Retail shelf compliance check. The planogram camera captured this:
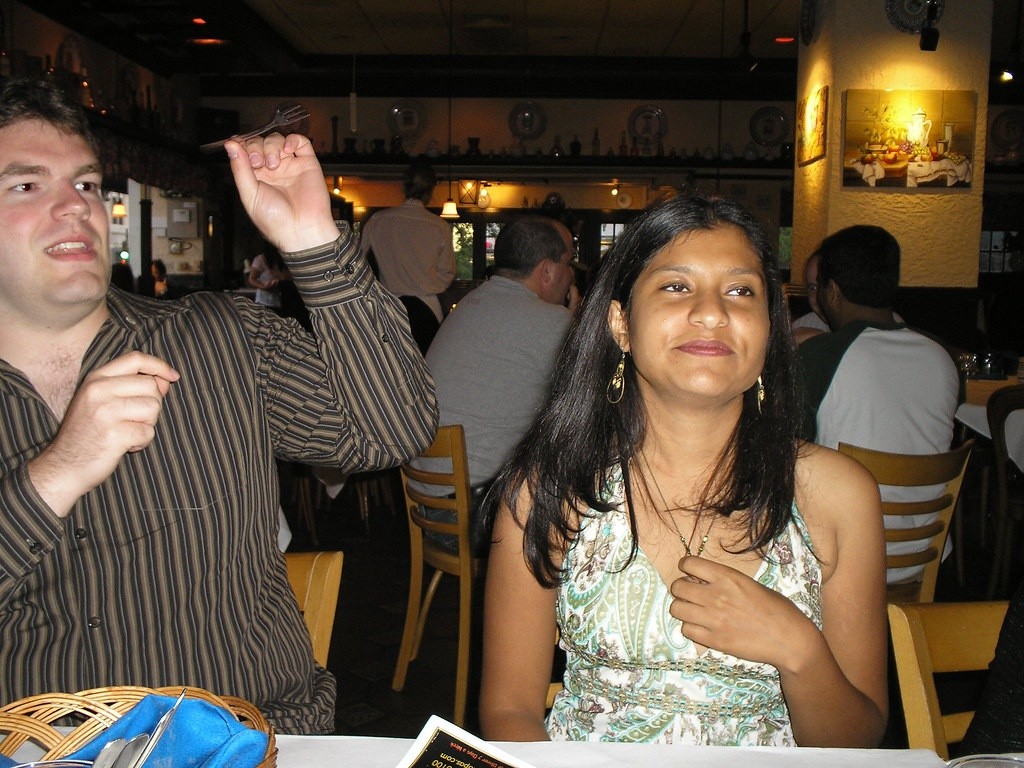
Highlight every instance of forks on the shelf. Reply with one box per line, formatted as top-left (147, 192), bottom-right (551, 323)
top-left (199, 104), bottom-right (311, 152)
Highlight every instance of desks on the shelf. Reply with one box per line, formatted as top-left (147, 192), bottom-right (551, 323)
top-left (0, 734), bottom-right (951, 768)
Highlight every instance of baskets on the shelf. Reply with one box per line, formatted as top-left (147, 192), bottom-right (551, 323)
top-left (0, 687), bottom-right (279, 768)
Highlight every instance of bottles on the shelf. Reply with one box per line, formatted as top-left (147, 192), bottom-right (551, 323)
top-left (670, 147), bottom-right (676, 160)
top-left (571, 134), bottom-right (581, 158)
top-left (552, 135), bottom-right (561, 162)
top-left (619, 130), bottom-right (628, 159)
top-left (959, 351), bottom-right (980, 397)
top-left (655, 134), bottom-right (663, 161)
top-left (591, 128), bottom-right (600, 158)
top-left (426, 140), bottom-right (440, 158)
top-left (694, 147), bottom-right (700, 160)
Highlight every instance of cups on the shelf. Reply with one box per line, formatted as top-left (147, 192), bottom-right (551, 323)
top-left (944, 754), bottom-right (1024, 768)
top-left (9, 760), bottom-right (95, 768)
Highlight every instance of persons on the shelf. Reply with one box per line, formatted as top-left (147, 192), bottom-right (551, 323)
top-left (150, 260), bottom-right (178, 299)
top-left (0, 67), bottom-right (443, 737)
top-left (955, 583), bottom-right (1024, 756)
top-left (482, 193), bottom-right (890, 749)
top-left (789, 224), bottom-right (961, 597)
top-left (247, 158), bottom-right (584, 555)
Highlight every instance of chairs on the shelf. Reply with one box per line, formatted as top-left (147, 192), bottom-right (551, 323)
top-left (280, 550), bottom-right (346, 667)
top-left (390, 422), bottom-right (493, 730)
top-left (883, 594), bottom-right (1011, 755)
top-left (835, 438), bottom-right (982, 604)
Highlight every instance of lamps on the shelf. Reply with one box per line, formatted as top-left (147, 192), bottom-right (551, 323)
top-left (110, 192), bottom-right (128, 219)
top-left (442, 0), bottom-right (460, 220)
top-left (735, 0), bottom-right (760, 72)
top-left (331, 176), bottom-right (344, 195)
top-left (610, 182), bottom-right (622, 196)
top-left (918, 0), bottom-right (941, 52)
top-left (346, 50), bottom-right (360, 133)
top-left (478, 182), bottom-right (495, 208)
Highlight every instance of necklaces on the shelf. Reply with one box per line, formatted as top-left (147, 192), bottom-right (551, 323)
top-left (640, 444), bottom-right (725, 583)
